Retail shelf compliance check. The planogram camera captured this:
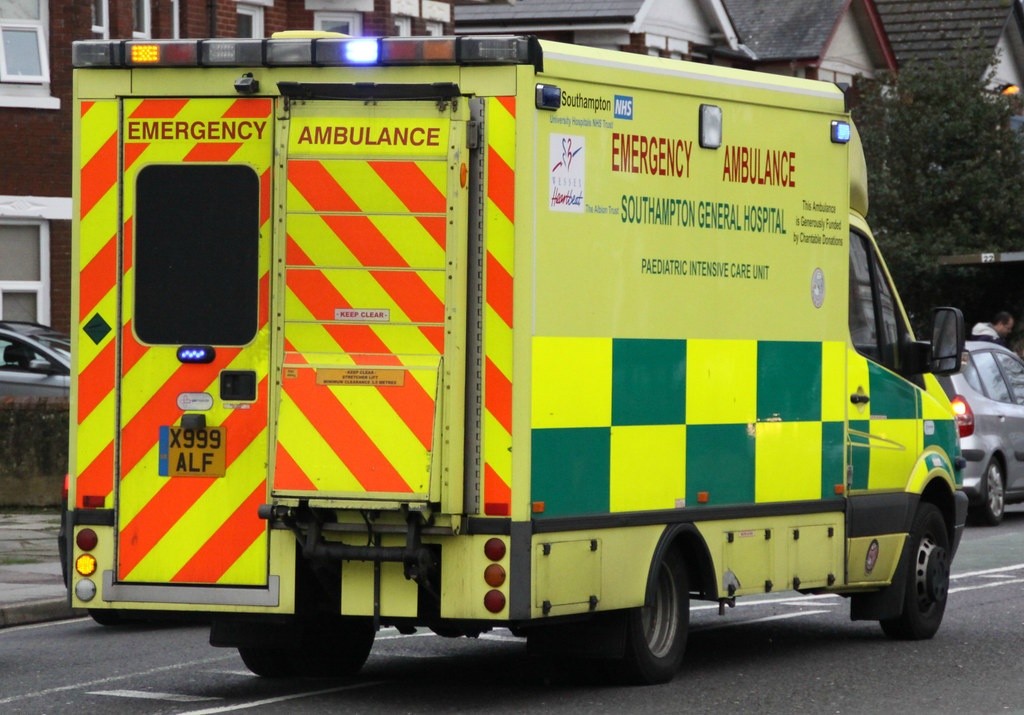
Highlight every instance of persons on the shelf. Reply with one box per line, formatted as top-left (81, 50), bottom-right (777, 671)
top-left (970, 310), bottom-right (1014, 345)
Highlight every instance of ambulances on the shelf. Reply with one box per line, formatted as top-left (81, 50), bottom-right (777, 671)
top-left (58, 21), bottom-right (971, 687)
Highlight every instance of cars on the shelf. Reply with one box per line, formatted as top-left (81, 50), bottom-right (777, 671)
top-left (912, 340), bottom-right (1023, 528)
top-left (1, 318), bottom-right (71, 401)
